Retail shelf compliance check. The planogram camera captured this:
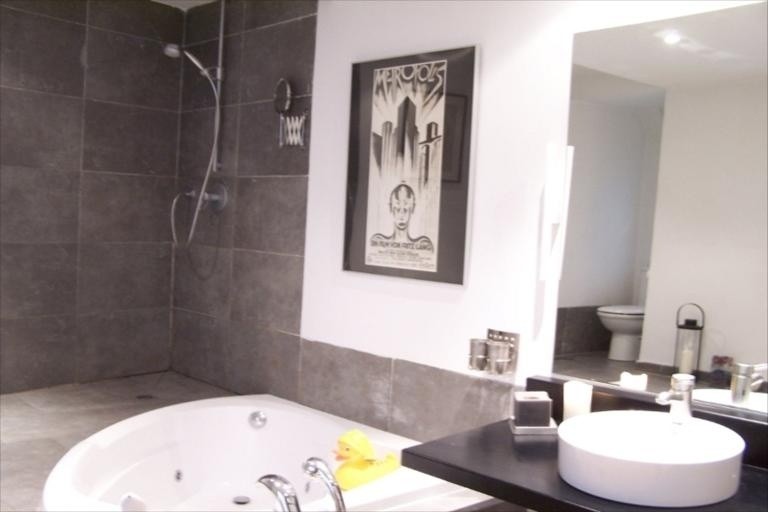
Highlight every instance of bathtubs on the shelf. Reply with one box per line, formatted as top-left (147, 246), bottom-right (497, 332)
top-left (42, 393), bottom-right (495, 512)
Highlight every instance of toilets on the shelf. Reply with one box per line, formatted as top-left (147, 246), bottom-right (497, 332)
top-left (597, 268), bottom-right (650, 362)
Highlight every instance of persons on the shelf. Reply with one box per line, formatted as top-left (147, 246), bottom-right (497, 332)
top-left (369, 185), bottom-right (434, 253)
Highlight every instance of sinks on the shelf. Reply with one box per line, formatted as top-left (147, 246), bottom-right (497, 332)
top-left (558, 411), bottom-right (747, 508)
top-left (692, 388), bottom-right (768, 426)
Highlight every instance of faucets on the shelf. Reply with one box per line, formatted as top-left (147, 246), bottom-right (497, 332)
top-left (730, 364), bottom-right (759, 402)
top-left (653, 373), bottom-right (695, 418)
top-left (301, 456), bottom-right (346, 512)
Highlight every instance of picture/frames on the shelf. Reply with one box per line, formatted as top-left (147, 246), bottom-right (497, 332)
top-left (343, 44), bottom-right (479, 284)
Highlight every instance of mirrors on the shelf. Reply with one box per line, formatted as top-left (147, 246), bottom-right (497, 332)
top-left (550, 1), bottom-right (768, 413)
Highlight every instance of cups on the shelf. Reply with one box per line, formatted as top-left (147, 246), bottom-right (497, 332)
top-left (486, 341), bottom-right (510, 375)
top-left (470, 339), bottom-right (488, 370)
top-left (562, 380), bottom-right (593, 421)
